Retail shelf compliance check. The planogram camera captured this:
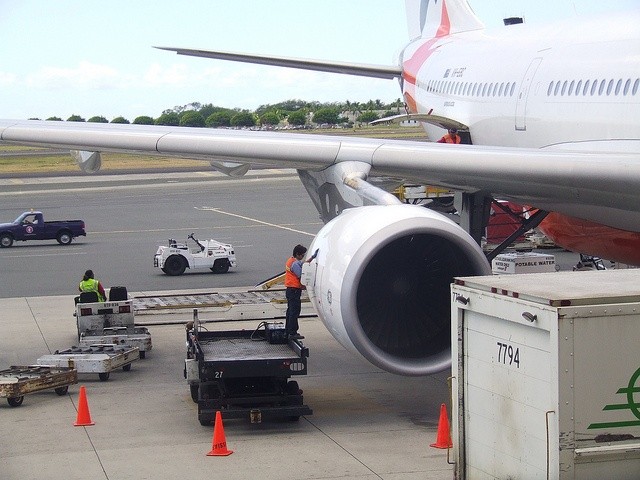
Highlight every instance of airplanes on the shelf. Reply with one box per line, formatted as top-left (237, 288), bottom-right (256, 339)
top-left (1, 1), bottom-right (639, 377)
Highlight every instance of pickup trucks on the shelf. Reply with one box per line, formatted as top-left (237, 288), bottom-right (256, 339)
top-left (0, 209), bottom-right (86, 248)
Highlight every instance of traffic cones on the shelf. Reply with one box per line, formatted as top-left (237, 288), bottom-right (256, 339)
top-left (429, 403), bottom-right (453, 449)
top-left (206, 411), bottom-right (233, 456)
top-left (72, 385), bottom-right (95, 426)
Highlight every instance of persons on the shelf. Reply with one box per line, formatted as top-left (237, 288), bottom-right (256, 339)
top-left (437, 128), bottom-right (461, 144)
top-left (78, 269), bottom-right (106, 302)
top-left (283, 244), bottom-right (319, 341)
top-left (25, 216), bottom-right (39, 225)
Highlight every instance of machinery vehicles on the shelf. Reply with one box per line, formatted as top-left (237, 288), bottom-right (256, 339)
top-left (183, 310), bottom-right (312, 425)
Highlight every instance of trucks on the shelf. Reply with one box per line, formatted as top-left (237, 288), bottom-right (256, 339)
top-left (446, 267), bottom-right (640, 480)
